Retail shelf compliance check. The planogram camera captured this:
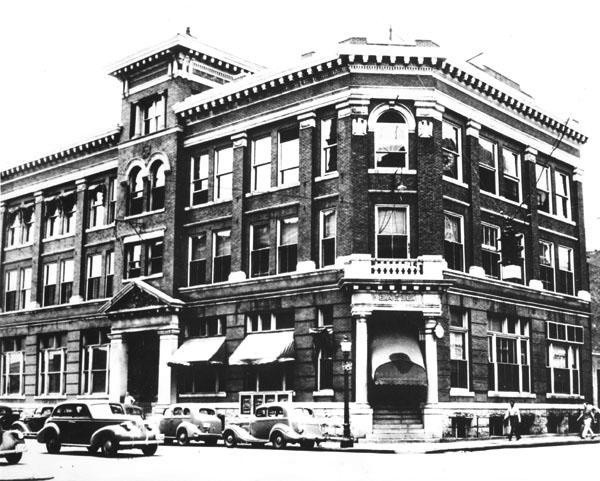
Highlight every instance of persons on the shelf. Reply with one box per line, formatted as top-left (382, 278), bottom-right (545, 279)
top-left (504, 400), bottom-right (522, 441)
top-left (576, 401), bottom-right (597, 439)
top-left (124, 392), bottom-right (136, 405)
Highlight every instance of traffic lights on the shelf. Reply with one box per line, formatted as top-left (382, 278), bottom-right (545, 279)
top-left (496, 228), bottom-right (507, 266)
top-left (508, 228), bottom-right (525, 266)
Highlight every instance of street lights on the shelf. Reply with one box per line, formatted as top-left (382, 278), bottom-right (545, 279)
top-left (339, 335), bottom-right (354, 448)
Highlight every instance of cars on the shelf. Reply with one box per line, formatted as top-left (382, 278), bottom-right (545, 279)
top-left (0, 399), bottom-right (56, 465)
top-left (222, 401), bottom-right (328, 450)
top-left (158, 403), bottom-right (223, 446)
top-left (35, 399), bottom-right (165, 458)
